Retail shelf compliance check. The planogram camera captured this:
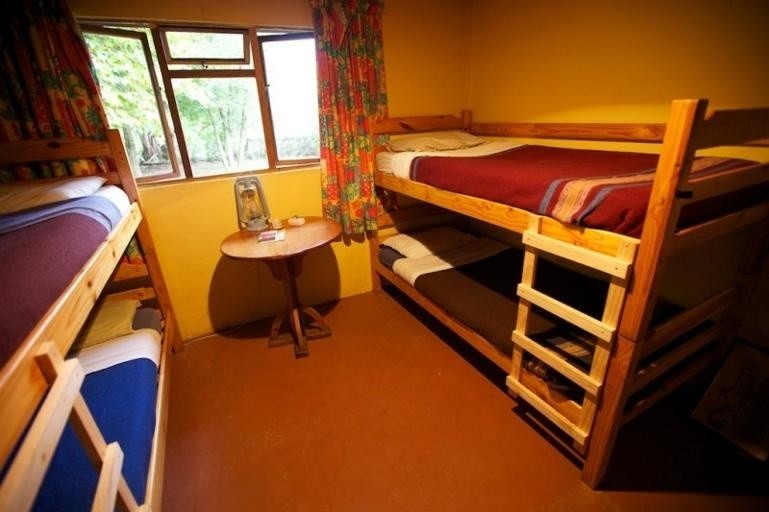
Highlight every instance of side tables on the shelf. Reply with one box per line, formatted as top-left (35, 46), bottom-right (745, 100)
top-left (220, 215), bottom-right (345, 359)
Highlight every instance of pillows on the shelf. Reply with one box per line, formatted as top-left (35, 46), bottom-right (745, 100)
top-left (383, 129), bottom-right (488, 153)
top-left (67, 297), bottom-right (142, 345)
top-left (1, 174), bottom-right (108, 215)
top-left (380, 225), bottom-right (478, 260)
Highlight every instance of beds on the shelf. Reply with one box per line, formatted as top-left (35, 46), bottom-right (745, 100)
top-left (0, 123), bottom-right (187, 512)
top-left (367, 93), bottom-right (760, 490)
